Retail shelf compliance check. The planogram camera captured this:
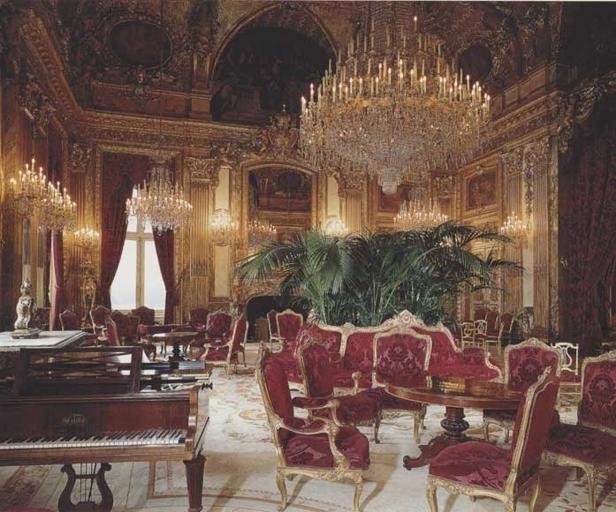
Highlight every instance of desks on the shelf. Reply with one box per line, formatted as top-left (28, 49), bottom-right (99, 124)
top-left (384, 372), bottom-right (527, 472)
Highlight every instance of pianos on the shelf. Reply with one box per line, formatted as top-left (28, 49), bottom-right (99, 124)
top-left (0, 345), bottom-right (213, 512)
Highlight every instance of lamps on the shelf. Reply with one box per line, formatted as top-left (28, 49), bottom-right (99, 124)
top-left (0, 102), bottom-right (278, 242)
top-left (294, 0), bottom-right (524, 240)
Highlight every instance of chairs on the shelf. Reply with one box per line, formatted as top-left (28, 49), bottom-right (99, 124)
top-left (260, 307), bottom-right (585, 405)
top-left (424, 359), bottom-right (562, 512)
top-left (0, 299), bottom-right (247, 385)
top-left (536, 349), bottom-right (616, 512)
top-left (254, 338), bottom-right (372, 511)
top-left (482, 336), bottom-right (560, 445)
top-left (296, 338), bottom-right (384, 446)
top-left (350, 324), bottom-right (435, 442)
